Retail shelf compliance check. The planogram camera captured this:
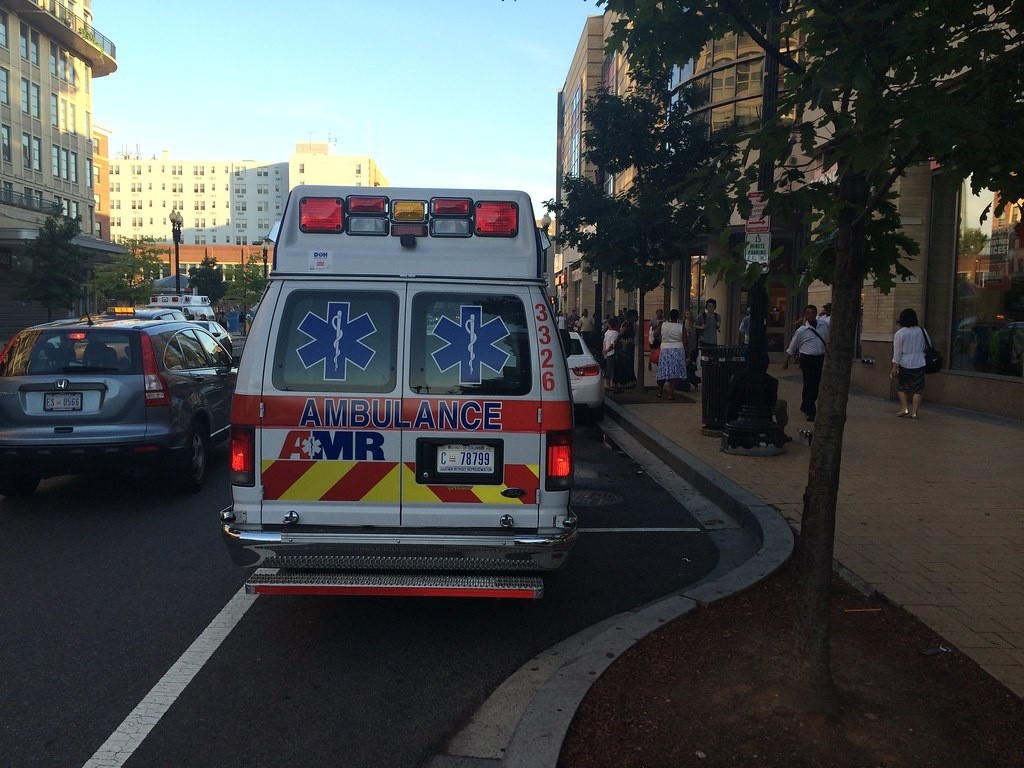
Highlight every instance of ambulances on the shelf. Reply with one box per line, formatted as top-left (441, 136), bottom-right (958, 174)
top-left (219, 186), bottom-right (575, 600)
top-left (150, 293), bottom-right (216, 322)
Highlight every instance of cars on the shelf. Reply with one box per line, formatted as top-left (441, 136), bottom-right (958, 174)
top-left (189, 320), bottom-right (233, 357)
top-left (568, 331), bottom-right (605, 424)
top-left (99, 306), bottom-right (187, 321)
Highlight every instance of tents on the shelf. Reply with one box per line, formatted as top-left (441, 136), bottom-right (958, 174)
top-left (154, 274), bottom-right (190, 288)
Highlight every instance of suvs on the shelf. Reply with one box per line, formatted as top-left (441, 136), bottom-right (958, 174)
top-left (0, 317), bottom-right (242, 501)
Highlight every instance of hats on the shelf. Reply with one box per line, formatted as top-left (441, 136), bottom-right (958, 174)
top-left (823, 303), bottom-right (831, 309)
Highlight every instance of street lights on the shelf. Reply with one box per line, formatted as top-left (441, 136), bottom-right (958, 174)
top-left (541, 213), bottom-right (551, 276)
top-left (261, 239), bottom-right (268, 279)
top-left (168, 210), bottom-right (184, 296)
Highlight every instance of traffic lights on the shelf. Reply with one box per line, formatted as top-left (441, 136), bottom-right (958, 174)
top-left (550, 296), bottom-right (555, 305)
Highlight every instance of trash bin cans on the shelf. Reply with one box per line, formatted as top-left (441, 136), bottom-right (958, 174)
top-left (696, 344), bottom-right (755, 437)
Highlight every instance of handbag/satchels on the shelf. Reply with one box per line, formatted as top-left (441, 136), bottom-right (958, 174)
top-left (924, 348), bottom-right (944, 374)
top-left (649, 327), bottom-right (655, 345)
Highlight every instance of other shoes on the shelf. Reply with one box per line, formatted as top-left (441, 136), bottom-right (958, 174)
top-left (656, 390), bottom-right (662, 397)
top-left (647, 359), bottom-right (652, 371)
top-left (897, 408), bottom-right (909, 417)
top-left (911, 414), bottom-right (919, 419)
top-left (605, 383), bottom-right (618, 390)
top-left (800, 407), bottom-right (815, 421)
top-left (668, 394), bottom-right (674, 399)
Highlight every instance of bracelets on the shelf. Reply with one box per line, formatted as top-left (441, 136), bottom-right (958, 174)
top-left (893, 367), bottom-right (897, 368)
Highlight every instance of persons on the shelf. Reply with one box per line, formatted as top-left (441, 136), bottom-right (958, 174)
top-left (683, 309), bottom-right (699, 366)
top-left (694, 299), bottom-right (721, 347)
top-left (215, 312), bottom-right (227, 331)
top-left (199, 313), bottom-right (214, 321)
top-left (889, 308), bottom-right (933, 419)
top-left (648, 308), bottom-right (668, 371)
top-left (603, 309), bottom-right (638, 386)
top-left (239, 311), bottom-right (245, 336)
top-left (555, 307), bottom-right (638, 391)
top-left (577, 309), bottom-right (596, 352)
top-left (783, 303), bottom-right (833, 422)
top-left (656, 309), bottom-right (688, 400)
top-left (603, 315), bottom-right (620, 391)
top-left (739, 304), bottom-right (752, 345)
